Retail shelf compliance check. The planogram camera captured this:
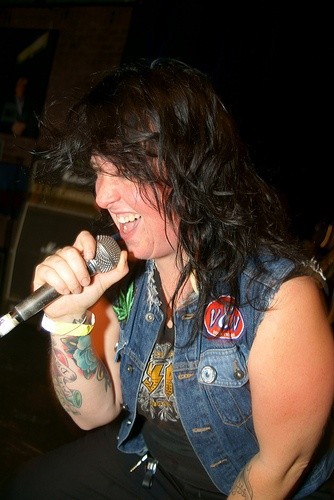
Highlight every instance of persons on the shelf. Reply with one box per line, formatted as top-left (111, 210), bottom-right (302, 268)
top-left (0, 56), bottom-right (334, 500)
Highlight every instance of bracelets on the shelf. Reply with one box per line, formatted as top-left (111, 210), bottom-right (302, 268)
top-left (41, 312), bottom-right (95, 336)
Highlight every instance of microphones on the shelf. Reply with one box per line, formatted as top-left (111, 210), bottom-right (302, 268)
top-left (0, 234), bottom-right (121, 339)
